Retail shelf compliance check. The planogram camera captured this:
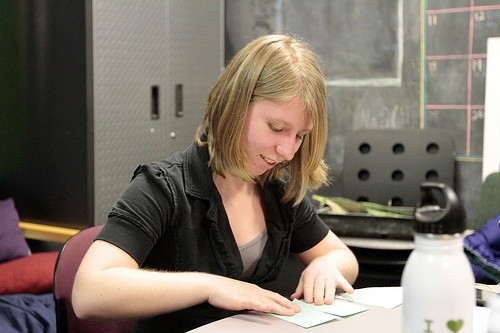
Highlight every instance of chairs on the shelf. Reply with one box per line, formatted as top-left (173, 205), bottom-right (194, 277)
top-left (53, 225), bottom-right (140, 333)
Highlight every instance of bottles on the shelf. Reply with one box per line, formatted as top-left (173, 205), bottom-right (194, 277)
top-left (400, 182), bottom-right (477, 333)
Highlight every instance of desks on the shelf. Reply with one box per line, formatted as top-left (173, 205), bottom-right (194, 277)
top-left (184, 283), bottom-right (499, 333)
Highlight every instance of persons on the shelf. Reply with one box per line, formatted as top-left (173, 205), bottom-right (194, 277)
top-left (70, 34), bottom-right (360, 333)
top-left (464, 213), bottom-right (500, 285)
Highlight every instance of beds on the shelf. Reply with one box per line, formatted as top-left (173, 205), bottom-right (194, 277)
top-left (0, 197), bottom-right (62, 333)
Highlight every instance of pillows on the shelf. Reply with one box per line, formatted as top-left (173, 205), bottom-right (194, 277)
top-left (0, 249), bottom-right (62, 295)
top-left (0, 198), bottom-right (32, 261)
